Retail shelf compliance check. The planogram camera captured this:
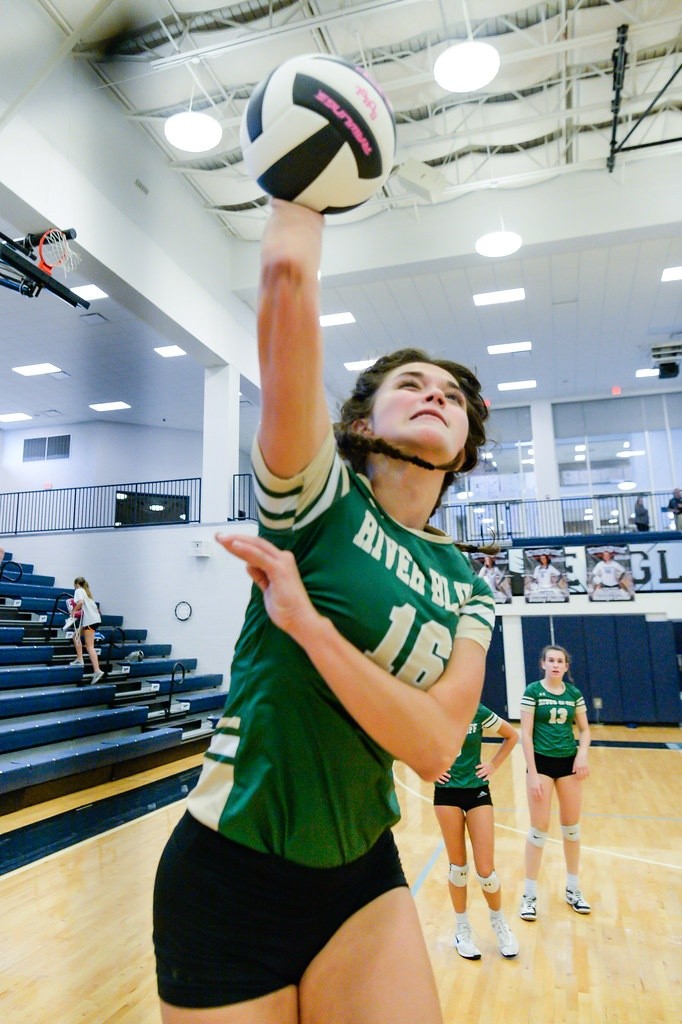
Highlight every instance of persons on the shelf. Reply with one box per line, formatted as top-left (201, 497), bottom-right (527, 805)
top-left (68, 577), bottom-right (105, 686)
top-left (589, 551), bottom-right (634, 602)
top-left (431, 701), bottom-right (521, 959)
top-left (150, 195), bottom-right (496, 1024)
top-left (524, 554), bottom-right (569, 602)
top-left (633, 496), bottom-right (650, 533)
top-left (666, 489), bottom-right (682, 533)
top-left (521, 646), bottom-right (591, 923)
top-left (478, 555), bottom-right (512, 604)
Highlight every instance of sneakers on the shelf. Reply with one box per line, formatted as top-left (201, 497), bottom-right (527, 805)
top-left (492, 914), bottom-right (519, 957)
top-left (565, 886), bottom-right (590, 914)
top-left (519, 894), bottom-right (537, 921)
top-left (452, 922), bottom-right (482, 960)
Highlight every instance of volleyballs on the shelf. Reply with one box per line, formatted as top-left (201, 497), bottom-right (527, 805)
top-left (241, 51), bottom-right (398, 217)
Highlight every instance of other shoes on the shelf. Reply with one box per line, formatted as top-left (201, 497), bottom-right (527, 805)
top-left (63, 618), bottom-right (78, 630)
top-left (91, 670), bottom-right (104, 685)
top-left (69, 658), bottom-right (84, 665)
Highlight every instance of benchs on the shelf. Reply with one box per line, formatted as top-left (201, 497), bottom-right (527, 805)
top-left (0, 628), bottom-right (183, 796)
top-left (0, 553), bottom-right (230, 729)
